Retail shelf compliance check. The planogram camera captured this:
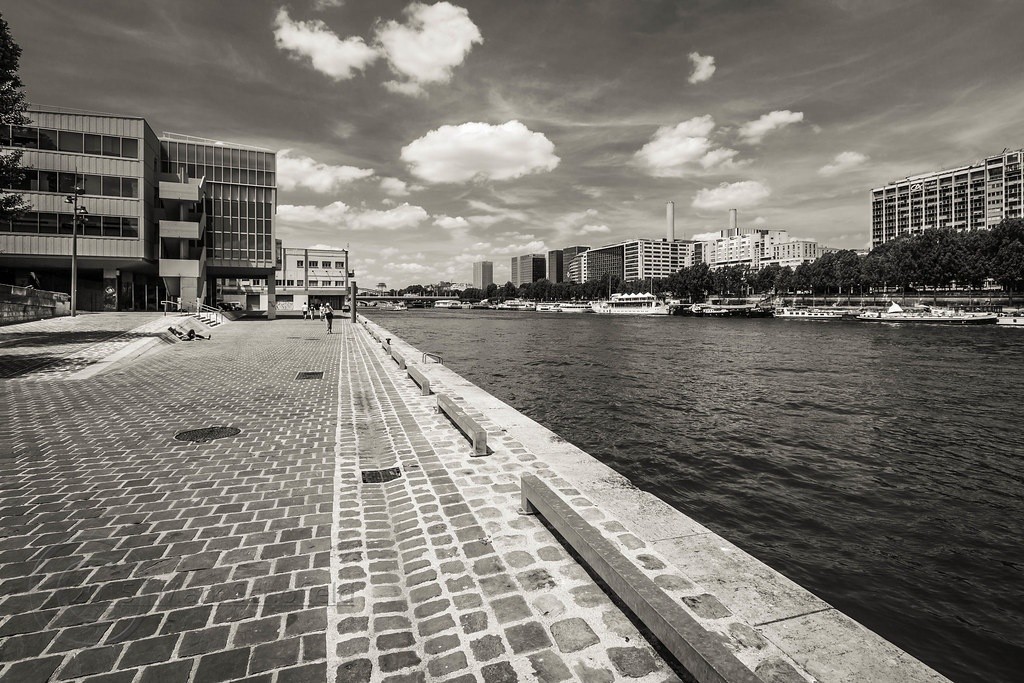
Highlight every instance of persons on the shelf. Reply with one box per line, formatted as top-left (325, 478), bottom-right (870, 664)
top-left (309, 303), bottom-right (316, 320)
top-left (319, 303), bottom-right (325, 321)
top-left (323, 303), bottom-right (334, 334)
top-left (301, 301), bottom-right (308, 320)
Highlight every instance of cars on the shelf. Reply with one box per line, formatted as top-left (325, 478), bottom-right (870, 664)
top-left (217, 302), bottom-right (243, 311)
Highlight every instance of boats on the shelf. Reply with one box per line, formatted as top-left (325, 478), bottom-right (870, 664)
top-left (379, 300), bottom-right (408, 311)
top-left (433, 292), bottom-right (1024, 325)
top-left (412, 300), bottom-right (431, 308)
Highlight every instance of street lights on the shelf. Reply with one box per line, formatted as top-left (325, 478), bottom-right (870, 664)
top-left (64, 166), bottom-right (88, 317)
